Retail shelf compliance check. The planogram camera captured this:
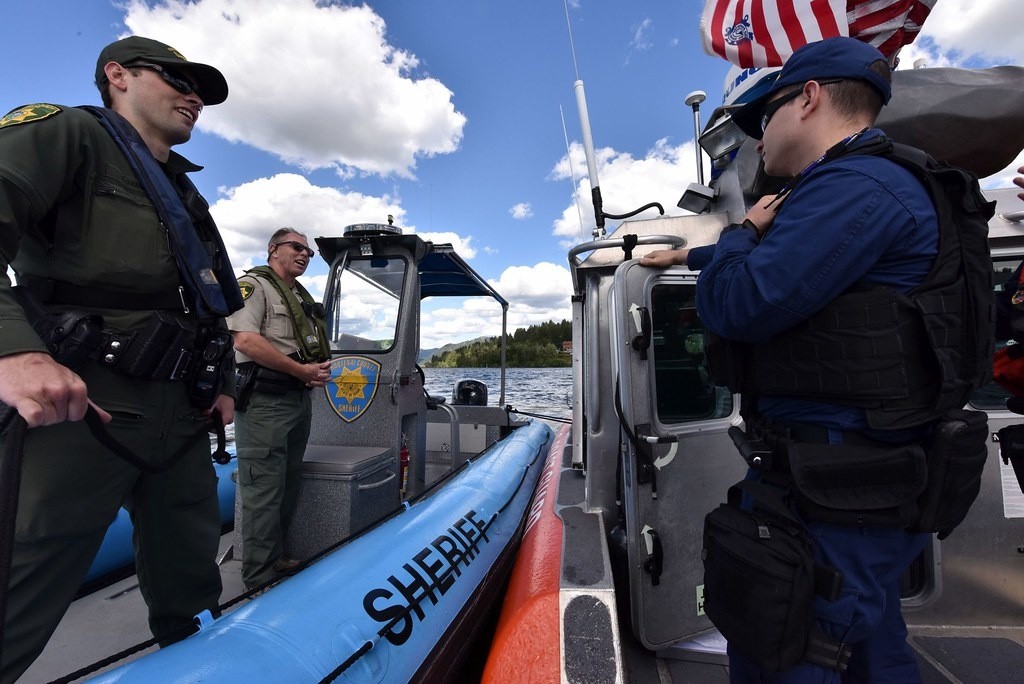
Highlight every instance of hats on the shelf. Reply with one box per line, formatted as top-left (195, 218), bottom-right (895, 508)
top-left (95, 35), bottom-right (227, 107)
top-left (730, 35), bottom-right (894, 140)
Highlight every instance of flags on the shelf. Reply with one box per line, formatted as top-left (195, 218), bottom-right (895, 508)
top-left (701, 1), bottom-right (937, 70)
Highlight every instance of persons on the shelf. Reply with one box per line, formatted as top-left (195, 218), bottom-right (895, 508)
top-left (639, 37), bottom-right (989, 684)
top-left (0, 36), bottom-right (245, 684)
top-left (225, 228), bottom-right (332, 600)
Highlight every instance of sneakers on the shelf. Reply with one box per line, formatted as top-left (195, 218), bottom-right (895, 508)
top-left (242, 555), bottom-right (315, 599)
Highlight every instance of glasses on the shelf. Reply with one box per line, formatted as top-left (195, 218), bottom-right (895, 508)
top-left (758, 80), bottom-right (840, 131)
top-left (275, 241), bottom-right (315, 258)
top-left (120, 63), bottom-right (208, 106)
